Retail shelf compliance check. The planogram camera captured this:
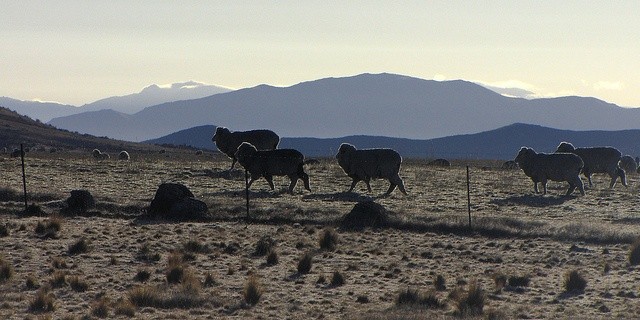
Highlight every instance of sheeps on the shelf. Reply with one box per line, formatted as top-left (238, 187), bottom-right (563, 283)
top-left (335, 143), bottom-right (409, 198)
top-left (234, 142), bottom-right (312, 195)
top-left (514, 146), bottom-right (585, 198)
top-left (551, 141), bottom-right (629, 190)
top-left (211, 126), bottom-right (280, 179)
